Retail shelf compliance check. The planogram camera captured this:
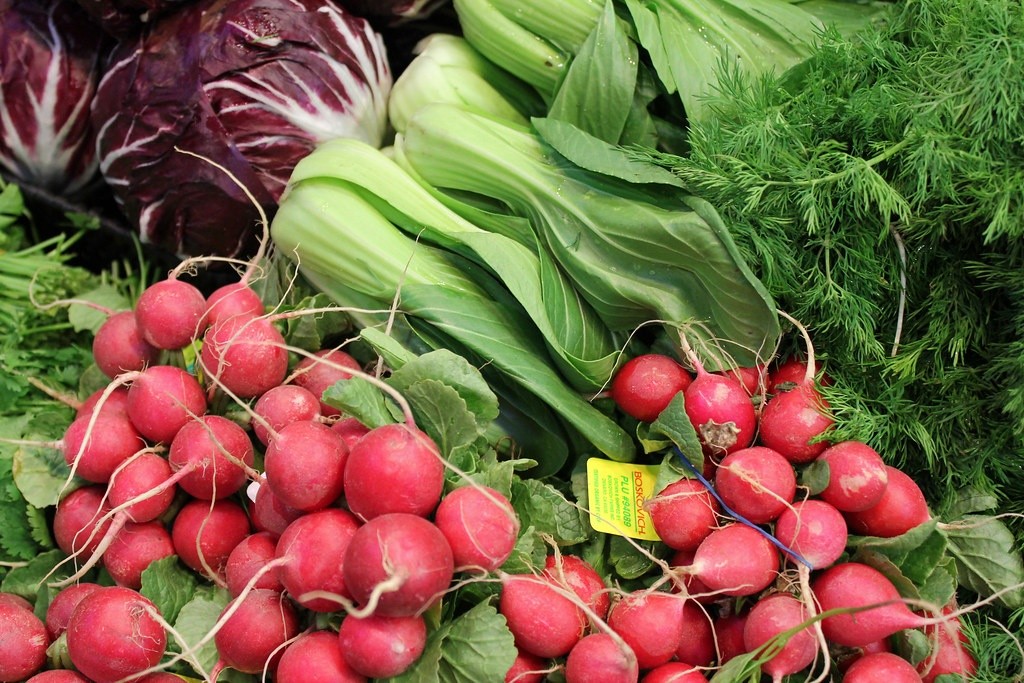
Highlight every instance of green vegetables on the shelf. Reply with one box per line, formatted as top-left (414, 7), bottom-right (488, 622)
top-left (266, 0), bottom-right (896, 470)
top-left (600, 0), bottom-right (1024, 683)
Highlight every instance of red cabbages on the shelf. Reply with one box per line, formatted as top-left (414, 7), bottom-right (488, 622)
top-left (0, 0), bottom-right (454, 297)
